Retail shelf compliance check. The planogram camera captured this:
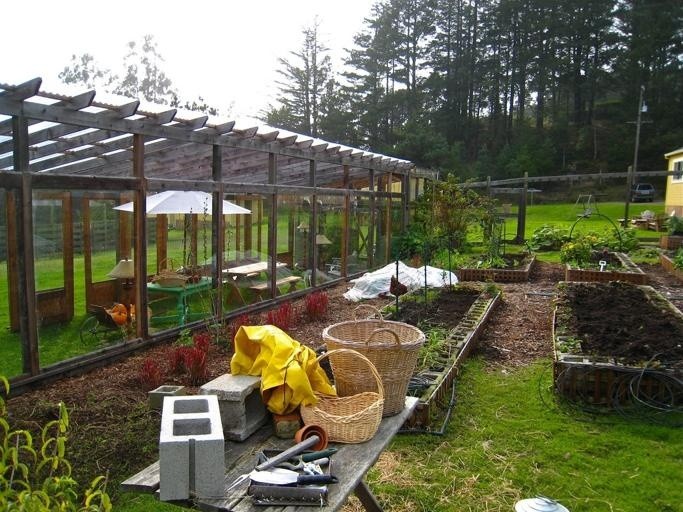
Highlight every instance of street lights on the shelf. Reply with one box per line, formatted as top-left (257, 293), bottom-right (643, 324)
top-left (296, 220), bottom-right (308, 268)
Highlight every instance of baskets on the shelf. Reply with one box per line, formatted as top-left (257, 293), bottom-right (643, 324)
top-left (321, 304), bottom-right (426, 418)
top-left (157, 258), bottom-right (180, 275)
top-left (299, 348), bottom-right (384, 444)
top-left (156, 274), bottom-right (185, 287)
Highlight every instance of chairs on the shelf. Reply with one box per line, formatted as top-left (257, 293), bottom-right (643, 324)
top-left (322, 250), bottom-right (363, 277)
top-left (80, 302), bottom-right (152, 345)
top-left (616, 209), bottom-right (678, 234)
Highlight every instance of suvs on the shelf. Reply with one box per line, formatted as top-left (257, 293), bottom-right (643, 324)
top-left (630, 181), bottom-right (655, 203)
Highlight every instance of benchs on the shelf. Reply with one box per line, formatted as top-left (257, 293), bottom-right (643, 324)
top-left (219, 272), bottom-right (259, 283)
top-left (248, 276), bottom-right (302, 303)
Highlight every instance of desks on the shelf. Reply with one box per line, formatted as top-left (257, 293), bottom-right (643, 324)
top-left (145, 276), bottom-right (214, 325)
top-left (220, 261), bottom-right (287, 306)
top-left (119, 384), bottom-right (418, 512)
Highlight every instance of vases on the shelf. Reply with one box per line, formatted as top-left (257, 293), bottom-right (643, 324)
top-left (303, 429), bottom-right (325, 451)
top-left (294, 423), bottom-right (329, 453)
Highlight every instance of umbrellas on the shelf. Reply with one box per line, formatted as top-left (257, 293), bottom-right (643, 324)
top-left (111, 190), bottom-right (253, 275)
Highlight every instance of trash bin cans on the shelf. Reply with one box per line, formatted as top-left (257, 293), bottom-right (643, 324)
top-left (502, 203), bottom-right (512, 216)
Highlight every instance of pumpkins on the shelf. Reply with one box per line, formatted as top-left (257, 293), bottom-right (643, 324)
top-left (112, 310), bottom-right (127, 324)
top-left (111, 304), bottom-right (127, 313)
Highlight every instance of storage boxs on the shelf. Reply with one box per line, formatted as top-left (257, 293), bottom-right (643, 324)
top-left (244, 446), bottom-right (333, 507)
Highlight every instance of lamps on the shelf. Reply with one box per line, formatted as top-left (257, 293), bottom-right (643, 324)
top-left (297, 221), bottom-right (310, 272)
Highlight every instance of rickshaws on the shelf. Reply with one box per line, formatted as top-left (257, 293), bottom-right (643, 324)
top-left (78, 298), bottom-right (169, 347)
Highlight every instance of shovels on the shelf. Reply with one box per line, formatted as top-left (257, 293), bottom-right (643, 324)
top-left (248, 469), bottom-right (340, 487)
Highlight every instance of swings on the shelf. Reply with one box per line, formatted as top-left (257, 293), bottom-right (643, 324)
top-left (577, 195), bottom-right (592, 219)
